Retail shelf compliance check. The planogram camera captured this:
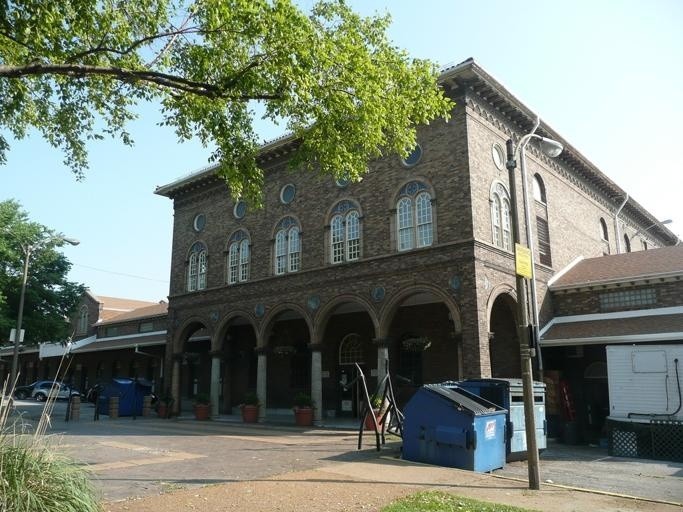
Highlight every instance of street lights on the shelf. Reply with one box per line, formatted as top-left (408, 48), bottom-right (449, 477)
top-left (506, 134), bottom-right (564, 488)
top-left (5, 224), bottom-right (80, 408)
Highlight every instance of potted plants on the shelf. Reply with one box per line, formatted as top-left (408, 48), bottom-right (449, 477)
top-left (243, 392), bottom-right (257, 423)
top-left (194, 393), bottom-right (209, 420)
top-left (155, 392), bottom-right (175, 417)
top-left (292, 389), bottom-right (315, 426)
top-left (364, 393), bottom-right (386, 431)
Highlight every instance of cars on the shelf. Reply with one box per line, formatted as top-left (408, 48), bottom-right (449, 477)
top-left (13, 380), bottom-right (84, 402)
